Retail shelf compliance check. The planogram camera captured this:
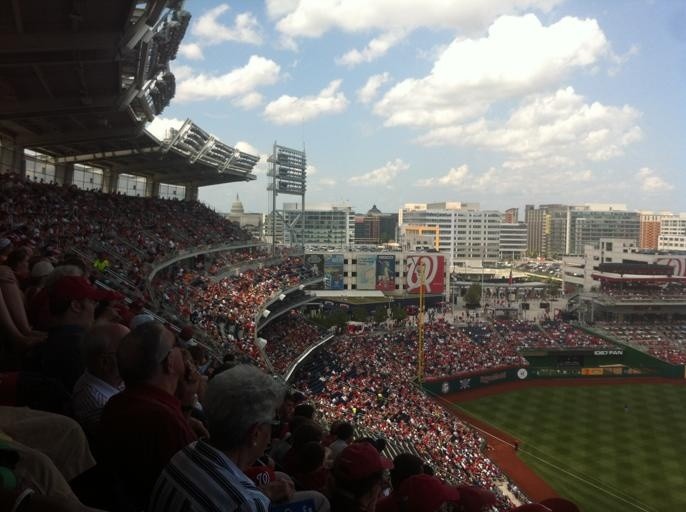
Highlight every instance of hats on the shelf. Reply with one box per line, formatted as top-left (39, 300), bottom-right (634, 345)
top-left (31, 260), bottom-right (155, 331)
top-left (352, 436), bottom-right (388, 455)
top-left (390, 452), bottom-right (434, 491)
top-left (331, 441), bottom-right (395, 484)
top-left (446, 486), bottom-right (497, 511)
top-left (407, 475), bottom-right (461, 511)
top-left (271, 392), bottom-right (354, 476)
top-left (509, 498), bottom-right (580, 512)
top-left (181, 326), bottom-right (193, 341)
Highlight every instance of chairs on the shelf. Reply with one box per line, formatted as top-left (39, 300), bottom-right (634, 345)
top-left (0, 172), bottom-right (528, 512)
top-left (526, 279), bottom-right (685, 375)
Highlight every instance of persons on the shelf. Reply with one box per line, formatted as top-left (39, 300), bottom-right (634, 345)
top-left (600, 281), bottom-right (685, 366)
top-left (3, 170), bottom-right (606, 512)
